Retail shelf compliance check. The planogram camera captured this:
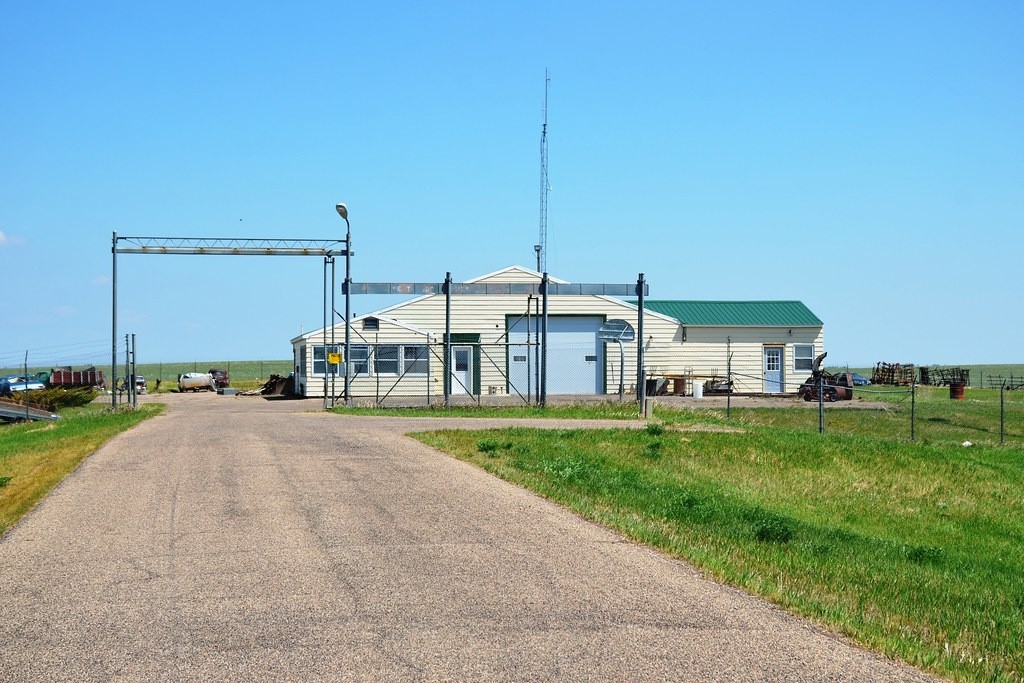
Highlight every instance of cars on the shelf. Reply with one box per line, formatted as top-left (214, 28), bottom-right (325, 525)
top-left (835, 371), bottom-right (871, 386)
top-left (0, 374), bottom-right (46, 394)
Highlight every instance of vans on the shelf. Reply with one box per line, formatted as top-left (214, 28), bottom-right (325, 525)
top-left (126, 374), bottom-right (147, 390)
top-left (209, 369), bottom-right (229, 386)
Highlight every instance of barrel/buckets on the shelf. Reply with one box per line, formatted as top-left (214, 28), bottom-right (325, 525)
top-left (950, 383), bottom-right (965, 400)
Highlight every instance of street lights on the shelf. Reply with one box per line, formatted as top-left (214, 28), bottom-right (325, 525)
top-left (335, 203), bottom-right (350, 403)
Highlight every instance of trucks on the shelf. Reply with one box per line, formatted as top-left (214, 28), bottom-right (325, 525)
top-left (34, 370), bottom-right (104, 389)
